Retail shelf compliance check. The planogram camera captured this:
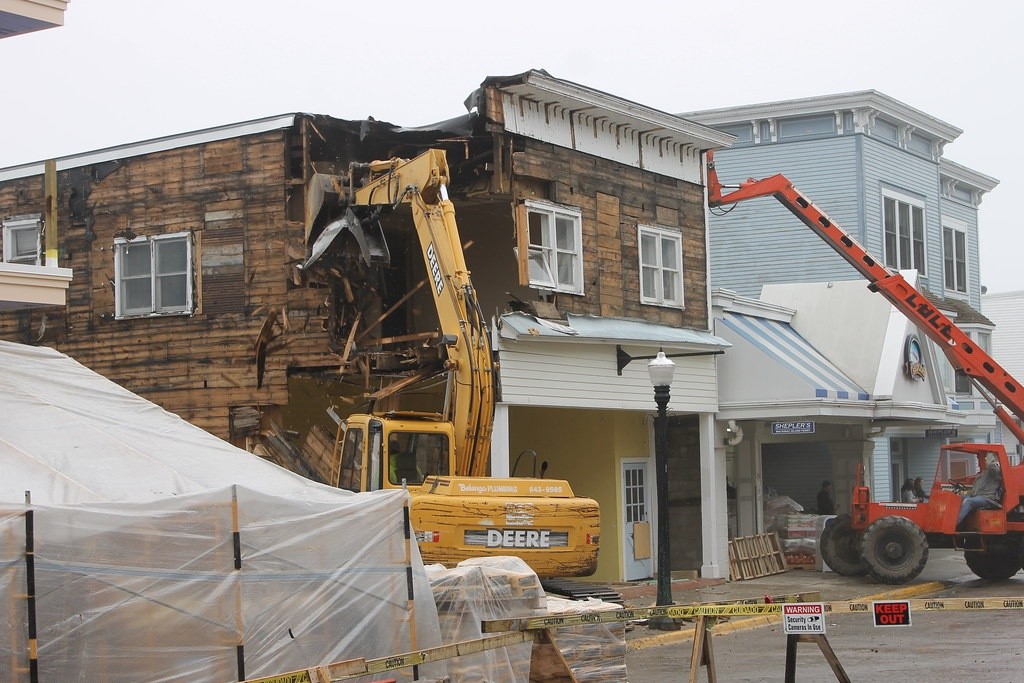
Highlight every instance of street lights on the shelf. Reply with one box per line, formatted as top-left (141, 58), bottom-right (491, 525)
top-left (646, 349), bottom-right (684, 631)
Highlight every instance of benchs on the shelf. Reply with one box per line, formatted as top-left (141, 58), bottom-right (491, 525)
top-left (966, 510), bottom-right (1006, 533)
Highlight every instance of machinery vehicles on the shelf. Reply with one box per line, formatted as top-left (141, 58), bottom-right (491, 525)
top-left (704, 150), bottom-right (1024, 586)
top-left (253, 148), bottom-right (636, 636)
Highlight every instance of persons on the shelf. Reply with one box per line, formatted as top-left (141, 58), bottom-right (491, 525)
top-left (388, 440), bottom-right (425, 486)
top-left (816, 479), bottom-right (836, 515)
top-left (901, 479), bottom-right (925, 504)
top-left (957, 451), bottom-right (1003, 530)
top-left (914, 477), bottom-right (930, 500)
